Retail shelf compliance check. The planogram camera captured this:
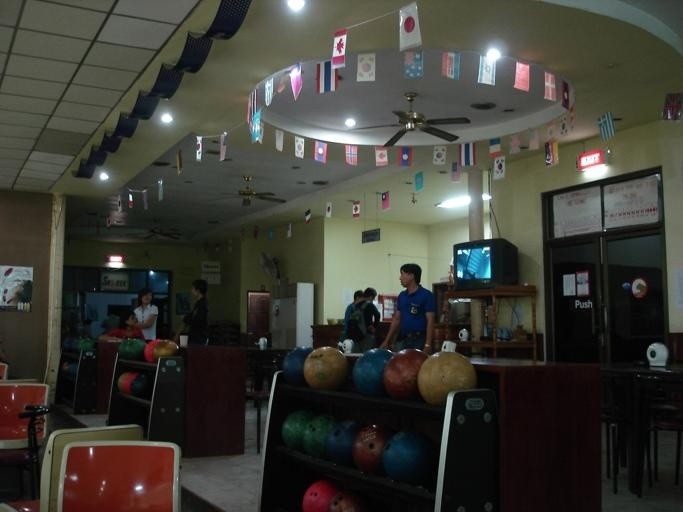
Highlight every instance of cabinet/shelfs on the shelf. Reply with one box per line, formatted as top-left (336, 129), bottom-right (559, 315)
top-left (444, 284), bottom-right (537, 360)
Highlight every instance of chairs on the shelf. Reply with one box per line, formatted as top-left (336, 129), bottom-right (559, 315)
top-left (594, 332), bottom-right (683, 499)
top-left (0, 363), bottom-right (183, 512)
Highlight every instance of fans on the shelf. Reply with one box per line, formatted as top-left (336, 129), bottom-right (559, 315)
top-left (208, 176), bottom-right (287, 207)
top-left (349, 92), bottom-right (470, 148)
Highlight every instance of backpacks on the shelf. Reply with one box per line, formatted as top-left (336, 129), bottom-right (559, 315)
top-left (348, 300), bottom-right (371, 342)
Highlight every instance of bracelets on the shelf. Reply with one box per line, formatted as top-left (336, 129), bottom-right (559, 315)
top-left (424, 343), bottom-right (431, 347)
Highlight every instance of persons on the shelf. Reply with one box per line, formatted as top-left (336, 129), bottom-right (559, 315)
top-left (379, 263), bottom-right (436, 355)
top-left (133, 287), bottom-right (159, 344)
top-left (339, 289), bottom-right (364, 353)
top-left (106, 309), bottom-right (145, 340)
top-left (182, 278), bottom-right (210, 344)
top-left (347, 287), bottom-right (381, 354)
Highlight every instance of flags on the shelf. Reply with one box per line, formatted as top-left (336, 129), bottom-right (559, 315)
top-left (661, 92), bottom-right (683, 120)
top-left (245, 50), bottom-right (578, 242)
top-left (597, 110), bottom-right (617, 144)
top-left (397, 1), bottom-right (422, 51)
top-left (112, 131), bottom-right (230, 215)
top-left (331, 29), bottom-right (346, 66)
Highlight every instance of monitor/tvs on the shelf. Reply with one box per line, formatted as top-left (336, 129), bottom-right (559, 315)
top-left (452, 238), bottom-right (519, 289)
